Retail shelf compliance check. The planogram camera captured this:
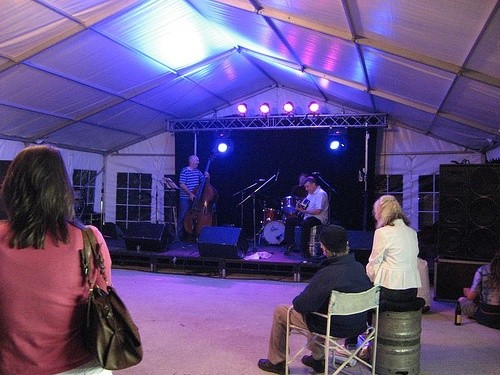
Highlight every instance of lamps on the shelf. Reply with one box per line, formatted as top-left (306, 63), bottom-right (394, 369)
top-left (237, 102), bottom-right (247, 117)
top-left (307, 101), bottom-right (319, 114)
top-left (259, 103), bottom-right (270, 117)
top-left (284, 102), bottom-right (293, 116)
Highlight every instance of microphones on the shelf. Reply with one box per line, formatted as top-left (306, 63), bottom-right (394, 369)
top-left (312, 172), bottom-right (319, 174)
top-left (275, 169), bottom-right (280, 181)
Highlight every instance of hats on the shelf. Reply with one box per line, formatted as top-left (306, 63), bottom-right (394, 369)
top-left (318, 224), bottom-right (348, 252)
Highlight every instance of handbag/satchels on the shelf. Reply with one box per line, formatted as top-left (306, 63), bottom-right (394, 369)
top-left (67, 219), bottom-right (143, 371)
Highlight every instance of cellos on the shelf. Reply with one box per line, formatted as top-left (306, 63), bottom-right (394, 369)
top-left (183, 145), bottom-right (219, 245)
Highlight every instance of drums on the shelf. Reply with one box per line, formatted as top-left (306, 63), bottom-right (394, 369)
top-left (263, 208), bottom-right (278, 226)
top-left (281, 196), bottom-right (300, 215)
top-left (263, 220), bottom-right (286, 245)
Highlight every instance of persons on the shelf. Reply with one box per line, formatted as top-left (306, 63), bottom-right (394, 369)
top-left (284, 177), bottom-right (329, 259)
top-left (0, 146), bottom-right (112, 375)
top-left (416, 247), bottom-right (432, 313)
top-left (174, 155), bottom-right (210, 242)
top-left (290, 173), bottom-right (309, 199)
top-left (258, 224), bottom-right (372, 375)
top-left (458, 250), bottom-right (500, 325)
top-left (331, 195), bottom-right (421, 357)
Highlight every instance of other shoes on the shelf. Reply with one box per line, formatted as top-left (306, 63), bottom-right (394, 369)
top-left (284, 244), bottom-right (296, 255)
top-left (301, 355), bottom-right (325, 373)
top-left (258, 358), bottom-right (290, 375)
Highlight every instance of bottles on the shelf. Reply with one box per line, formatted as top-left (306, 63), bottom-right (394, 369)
top-left (454, 301), bottom-right (462, 325)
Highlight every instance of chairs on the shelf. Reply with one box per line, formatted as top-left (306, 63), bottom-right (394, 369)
top-left (285, 284), bottom-right (381, 375)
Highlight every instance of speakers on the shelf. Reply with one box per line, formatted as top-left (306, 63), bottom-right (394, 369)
top-left (344, 230), bottom-right (373, 264)
top-left (438, 164), bottom-right (500, 261)
top-left (123, 222), bottom-right (169, 253)
top-left (106, 223), bottom-right (124, 239)
top-left (197, 225), bottom-right (249, 259)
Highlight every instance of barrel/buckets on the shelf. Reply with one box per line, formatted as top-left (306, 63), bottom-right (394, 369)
top-left (370, 297), bottom-right (422, 375)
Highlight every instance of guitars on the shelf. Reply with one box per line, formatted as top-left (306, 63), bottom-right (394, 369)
top-left (295, 198), bottom-right (310, 218)
